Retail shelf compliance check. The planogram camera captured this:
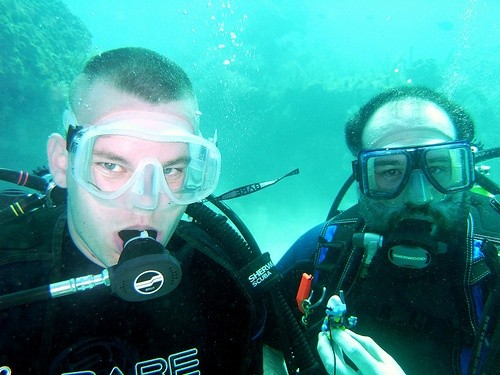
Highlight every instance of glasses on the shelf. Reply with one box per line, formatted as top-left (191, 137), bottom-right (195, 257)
top-left (353, 141), bottom-right (477, 201)
top-left (61, 108), bottom-right (224, 212)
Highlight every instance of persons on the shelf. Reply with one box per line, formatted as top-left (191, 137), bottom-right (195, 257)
top-left (263, 84), bottom-right (500, 375)
top-left (0, 48), bottom-right (288, 375)
top-left (321, 290), bottom-right (357, 340)
